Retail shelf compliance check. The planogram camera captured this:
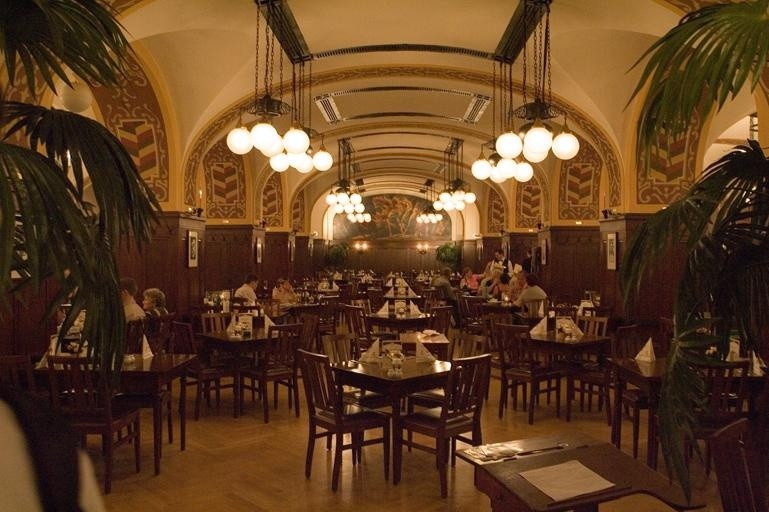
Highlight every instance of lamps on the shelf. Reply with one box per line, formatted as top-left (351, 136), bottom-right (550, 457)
top-left (431, 136), bottom-right (476, 213)
top-left (470, 0), bottom-right (581, 187)
top-left (413, 172), bottom-right (443, 223)
top-left (347, 210), bottom-right (372, 223)
top-left (321, 137), bottom-right (370, 214)
top-left (223, 1), bottom-right (336, 176)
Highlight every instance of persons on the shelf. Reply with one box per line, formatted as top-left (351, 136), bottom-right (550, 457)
top-left (429, 261), bottom-right (551, 316)
top-left (116, 276), bottom-right (145, 322)
top-left (139, 287), bottom-right (169, 319)
top-left (271, 277), bottom-right (296, 303)
top-left (234, 272), bottom-right (259, 306)
top-left (490, 247), bottom-right (515, 278)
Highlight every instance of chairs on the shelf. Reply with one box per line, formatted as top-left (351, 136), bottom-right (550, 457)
top-left (390, 348), bottom-right (496, 500)
top-left (1, 350), bottom-right (47, 403)
top-left (683, 349), bottom-right (752, 480)
top-left (291, 347), bottom-right (393, 493)
top-left (608, 318), bottom-right (666, 467)
top-left (39, 354), bottom-right (144, 496)
top-left (706, 415), bottom-right (769, 512)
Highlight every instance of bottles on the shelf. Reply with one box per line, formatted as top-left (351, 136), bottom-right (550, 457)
top-left (557, 320), bottom-right (578, 341)
top-left (231, 318), bottom-right (252, 340)
top-left (380, 351), bottom-right (407, 376)
